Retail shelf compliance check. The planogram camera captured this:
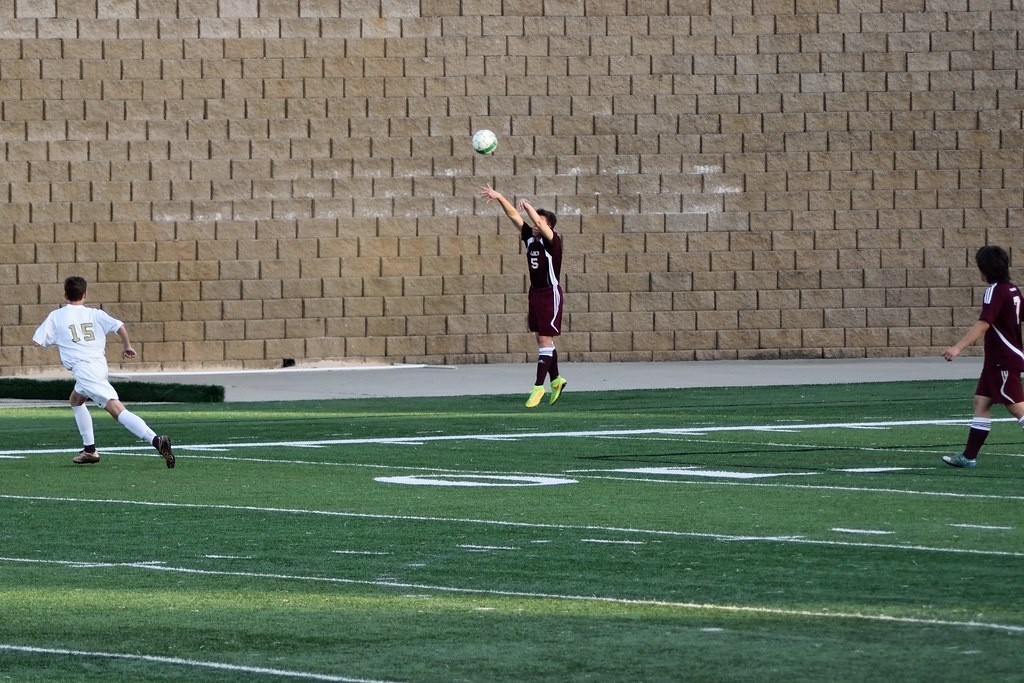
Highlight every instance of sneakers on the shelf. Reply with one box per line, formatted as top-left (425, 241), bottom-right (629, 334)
top-left (549, 374), bottom-right (567, 406)
top-left (73, 451), bottom-right (100, 464)
top-left (942, 453), bottom-right (976, 467)
top-left (157, 435), bottom-right (175, 468)
top-left (525, 385), bottom-right (545, 408)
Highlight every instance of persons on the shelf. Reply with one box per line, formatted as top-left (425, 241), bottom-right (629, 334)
top-left (478, 182), bottom-right (567, 408)
top-left (940, 246), bottom-right (1024, 468)
top-left (33, 276), bottom-right (176, 469)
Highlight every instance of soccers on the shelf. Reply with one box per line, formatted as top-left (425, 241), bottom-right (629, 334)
top-left (472, 128), bottom-right (500, 155)
